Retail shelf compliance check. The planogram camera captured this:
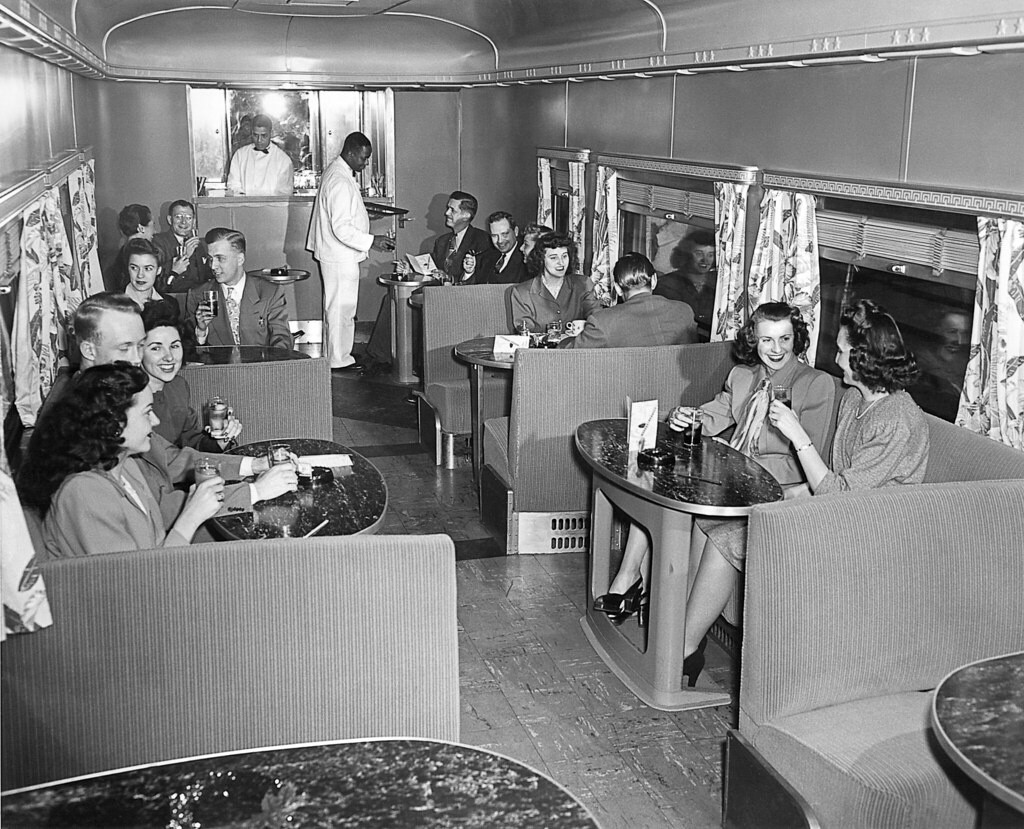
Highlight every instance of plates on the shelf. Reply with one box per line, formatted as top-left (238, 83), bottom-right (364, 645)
top-left (565, 330), bottom-right (575, 336)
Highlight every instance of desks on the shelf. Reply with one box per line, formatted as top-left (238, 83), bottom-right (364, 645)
top-left (185, 344), bottom-right (312, 365)
top-left (574, 418), bottom-right (786, 713)
top-left (931, 652), bottom-right (1024, 829)
top-left (456, 333), bottom-right (518, 490)
top-left (246, 269), bottom-right (310, 284)
top-left (379, 273), bottom-right (442, 386)
top-left (405, 294), bottom-right (425, 403)
top-left (0, 736), bottom-right (603, 829)
top-left (212, 438), bottom-right (389, 540)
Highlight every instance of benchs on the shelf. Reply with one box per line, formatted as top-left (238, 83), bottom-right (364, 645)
top-left (0, 285), bottom-right (1024, 829)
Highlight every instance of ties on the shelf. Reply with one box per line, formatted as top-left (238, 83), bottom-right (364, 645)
top-left (225, 286), bottom-right (240, 345)
top-left (449, 232), bottom-right (456, 252)
top-left (184, 238), bottom-right (189, 243)
top-left (495, 254), bottom-right (506, 272)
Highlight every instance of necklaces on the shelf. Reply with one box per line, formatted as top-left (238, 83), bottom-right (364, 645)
top-left (855, 393), bottom-right (889, 420)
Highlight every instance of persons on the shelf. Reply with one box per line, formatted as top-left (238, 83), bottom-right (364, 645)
top-left (306, 130), bottom-right (396, 380)
top-left (510, 231), bottom-right (604, 333)
top-left (18, 360), bottom-right (225, 560)
top-left (652, 229), bottom-right (718, 334)
top-left (225, 114), bottom-right (294, 198)
top-left (520, 221), bottom-right (556, 266)
top-left (682, 298), bottom-right (929, 688)
top-left (402, 212), bottom-right (532, 406)
top-left (358, 190), bottom-right (494, 381)
top-left (68, 291), bottom-right (302, 544)
top-left (112, 199), bottom-right (218, 294)
top-left (136, 298), bottom-right (243, 455)
top-left (906, 308), bottom-right (971, 423)
top-left (557, 251), bottom-right (699, 349)
top-left (185, 228), bottom-right (294, 350)
top-left (595, 302), bottom-right (837, 628)
top-left (120, 238), bottom-right (180, 332)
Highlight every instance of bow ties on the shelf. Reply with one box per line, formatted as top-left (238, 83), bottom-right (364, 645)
top-left (253, 145), bottom-right (271, 154)
top-left (352, 170), bottom-right (356, 177)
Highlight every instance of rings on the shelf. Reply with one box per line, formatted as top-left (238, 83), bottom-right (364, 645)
top-left (466, 261), bottom-right (468, 265)
top-left (218, 493), bottom-right (223, 500)
top-left (678, 407), bottom-right (680, 412)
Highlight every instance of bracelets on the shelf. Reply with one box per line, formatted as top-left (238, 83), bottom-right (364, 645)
top-left (796, 442), bottom-right (814, 453)
top-left (169, 271), bottom-right (180, 279)
top-left (197, 324), bottom-right (207, 331)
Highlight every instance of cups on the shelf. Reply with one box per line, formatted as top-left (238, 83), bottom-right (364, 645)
top-left (203, 291), bottom-right (219, 317)
top-left (682, 406), bottom-right (703, 447)
top-left (194, 459), bottom-right (221, 489)
top-left (566, 319), bottom-right (586, 334)
top-left (176, 246), bottom-right (186, 261)
top-left (186, 229), bottom-right (198, 240)
top-left (548, 322), bottom-right (561, 340)
top-left (268, 444), bottom-right (290, 468)
top-left (771, 386), bottom-right (792, 411)
top-left (442, 275), bottom-right (465, 285)
top-left (384, 231), bottom-right (396, 252)
top-left (208, 398), bottom-right (229, 439)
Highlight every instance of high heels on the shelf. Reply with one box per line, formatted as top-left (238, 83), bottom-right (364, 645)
top-left (683, 635), bottom-right (708, 687)
top-left (609, 591), bottom-right (650, 627)
top-left (593, 579), bottom-right (644, 613)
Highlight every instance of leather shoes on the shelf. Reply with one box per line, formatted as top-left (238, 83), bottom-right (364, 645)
top-left (351, 352), bottom-right (363, 360)
top-left (335, 362), bottom-right (366, 371)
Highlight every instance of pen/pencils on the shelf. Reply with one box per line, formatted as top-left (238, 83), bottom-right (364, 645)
top-left (677, 473), bottom-right (722, 485)
top-left (303, 519), bottom-right (329, 538)
